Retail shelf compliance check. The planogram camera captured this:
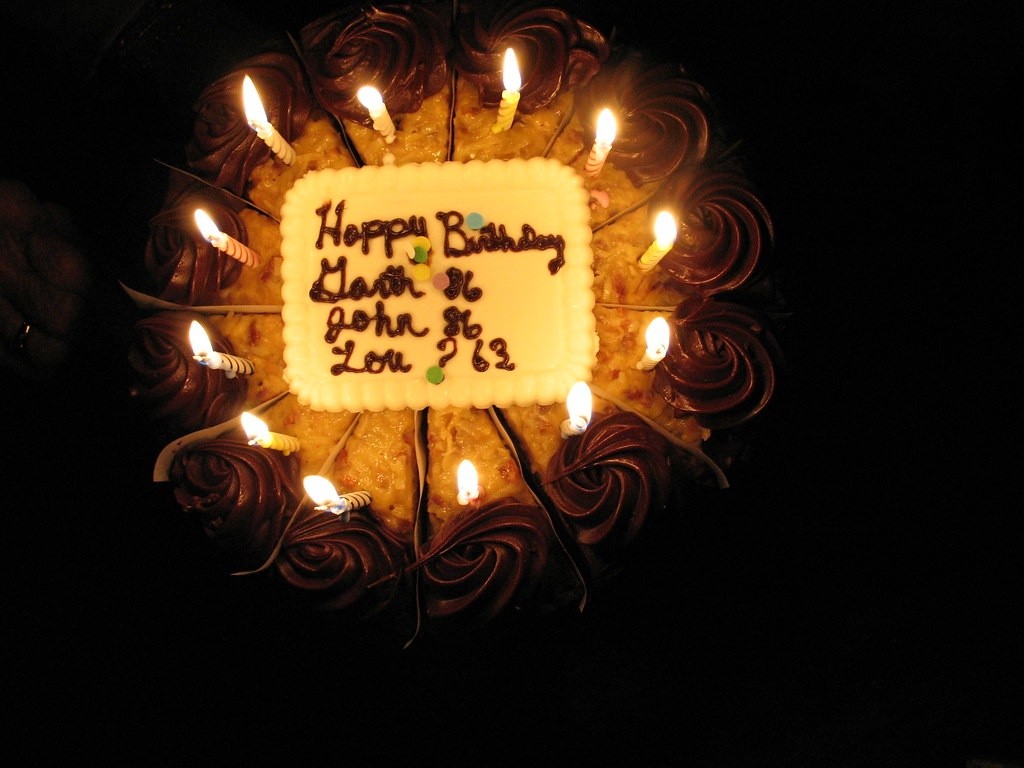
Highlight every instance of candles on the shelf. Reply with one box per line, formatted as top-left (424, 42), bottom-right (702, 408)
top-left (637, 210), bottom-right (677, 272)
top-left (303, 475), bottom-right (372, 516)
top-left (560, 381), bottom-right (593, 440)
top-left (194, 209), bottom-right (265, 268)
top-left (239, 412), bottom-right (301, 456)
top-left (492, 48), bottom-right (521, 134)
top-left (636, 317), bottom-right (670, 371)
top-left (456, 459), bottom-right (486, 506)
top-left (357, 86), bottom-right (396, 143)
top-left (242, 74), bottom-right (298, 165)
top-left (584, 108), bottom-right (618, 177)
top-left (189, 319), bottom-right (256, 374)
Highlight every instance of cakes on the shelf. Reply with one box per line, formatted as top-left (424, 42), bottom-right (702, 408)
top-left (120, 0), bottom-right (786, 644)
